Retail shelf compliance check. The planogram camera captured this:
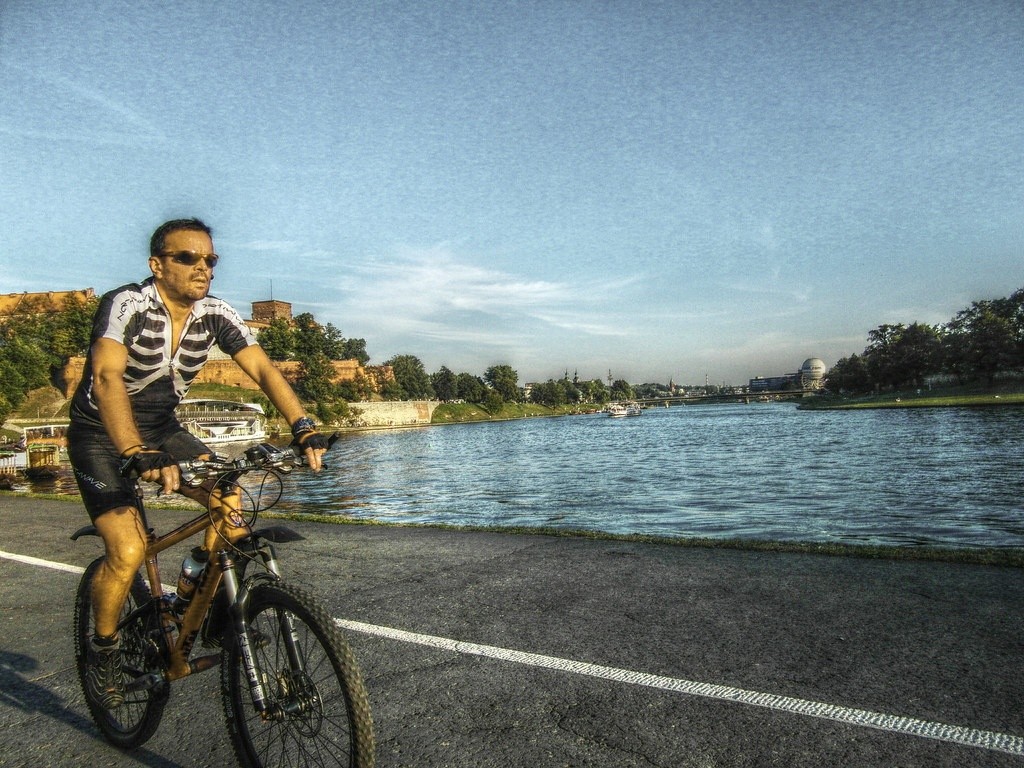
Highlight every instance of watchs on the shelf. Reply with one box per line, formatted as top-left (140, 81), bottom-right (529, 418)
top-left (291, 417), bottom-right (317, 437)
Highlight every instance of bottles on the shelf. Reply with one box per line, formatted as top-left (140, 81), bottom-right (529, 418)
top-left (175, 544), bottom-right (208, 615)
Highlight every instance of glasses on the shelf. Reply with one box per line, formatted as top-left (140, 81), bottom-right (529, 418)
top-left (156, 250), bottom-right (217, 269)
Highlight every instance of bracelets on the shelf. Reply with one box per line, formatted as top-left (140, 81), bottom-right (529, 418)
top-left (120, 444), bottom-right (149, 456)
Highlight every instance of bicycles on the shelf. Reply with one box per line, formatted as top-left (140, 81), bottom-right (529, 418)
top-left (67, 426), bottom-right (375, 768)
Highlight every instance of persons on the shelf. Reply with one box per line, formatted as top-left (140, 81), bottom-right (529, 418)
top-left (64, 216), bottom-right (332, 712)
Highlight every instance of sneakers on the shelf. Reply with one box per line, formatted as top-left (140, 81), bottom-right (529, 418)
top-left (84, 638), bottom-right (124, 710)
top-left (200, 616), bottom-right (271, 649)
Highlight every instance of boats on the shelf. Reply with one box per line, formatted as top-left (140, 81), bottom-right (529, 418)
top-left (608, 400), bottom-right (644, 418)
top-left (172, 398), bottom-right (266, 444)
top-left (25, 441), bottom-right (63, 479)
